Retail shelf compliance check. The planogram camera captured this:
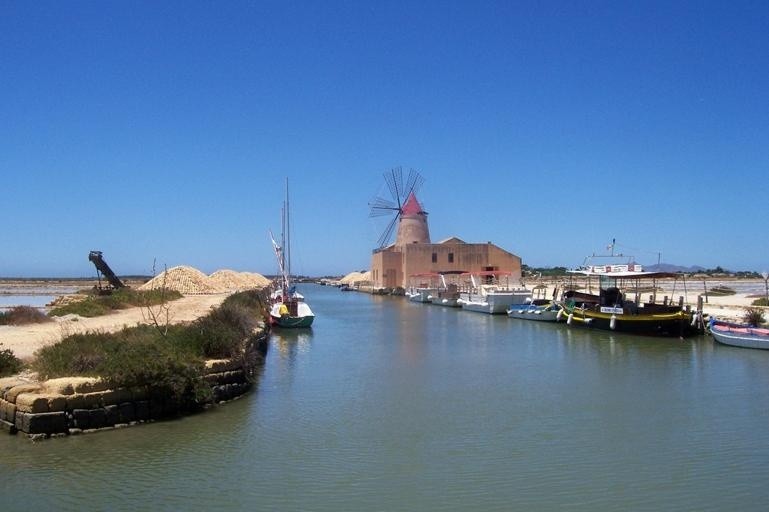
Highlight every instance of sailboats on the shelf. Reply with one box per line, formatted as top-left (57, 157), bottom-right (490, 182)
top-left (268, 178), bottom-right (315, 329)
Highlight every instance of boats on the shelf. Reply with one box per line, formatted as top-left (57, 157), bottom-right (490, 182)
top-left (505, 284), bottom-right (564, 321)
top-left (707, 315), bottom-right (769, 353)
top-left (555, 238), bottom-right (708, 340)
top-left (457, 270), bottom-right (533, 315)
top-left (427, 269), bottom-right (468, 307)
top-left (405, 272), bottom-right (438, 303)
